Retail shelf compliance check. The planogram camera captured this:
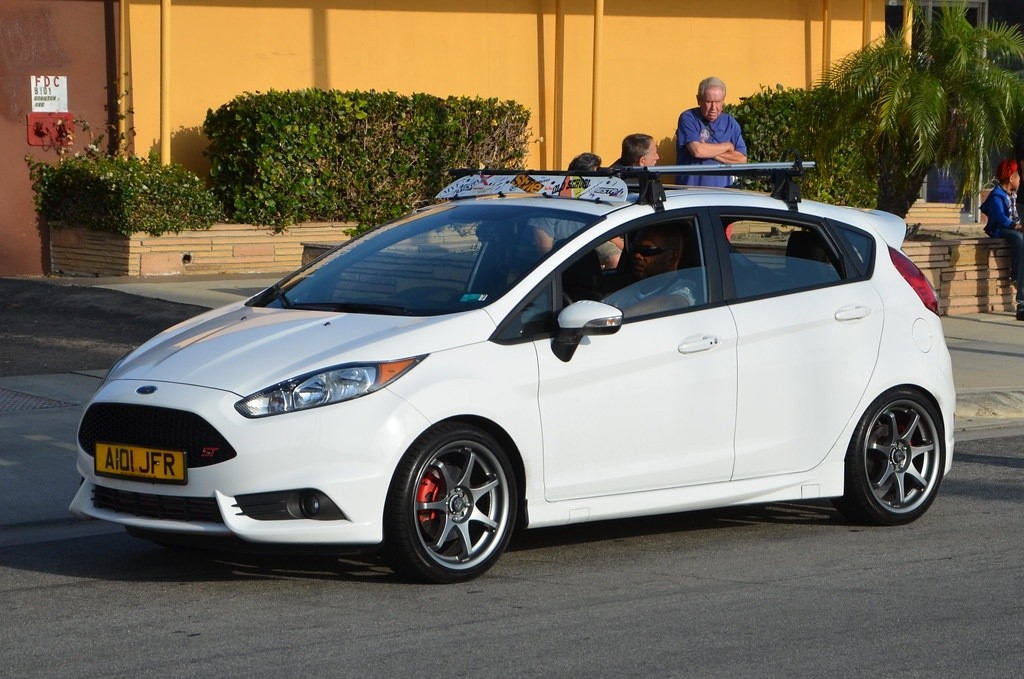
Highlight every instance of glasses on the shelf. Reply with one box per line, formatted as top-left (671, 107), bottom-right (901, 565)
top-left (628, 242), bottom-right (675, 256)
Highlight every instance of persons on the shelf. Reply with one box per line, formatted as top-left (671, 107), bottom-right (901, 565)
top-left (675, 78), bottom-right (748, 251)
top-left (522, 135), bottom-right (666, 278)
top-left (982, 159), bottom-right (1023, 321)
top-left (542, 222), bottom-right (704, 332)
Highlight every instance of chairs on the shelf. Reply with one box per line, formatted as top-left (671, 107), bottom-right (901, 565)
top-left (784, 230), bottom-right (835, 285)
top-left (551, 239), bottom-right (604, 300)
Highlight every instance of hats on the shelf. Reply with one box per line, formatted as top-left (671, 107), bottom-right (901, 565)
top-left (996, 160), bottom-right (1018, 182)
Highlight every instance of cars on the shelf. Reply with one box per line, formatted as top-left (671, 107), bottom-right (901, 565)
top-left (69, 159), bottom-right (956, 583)
